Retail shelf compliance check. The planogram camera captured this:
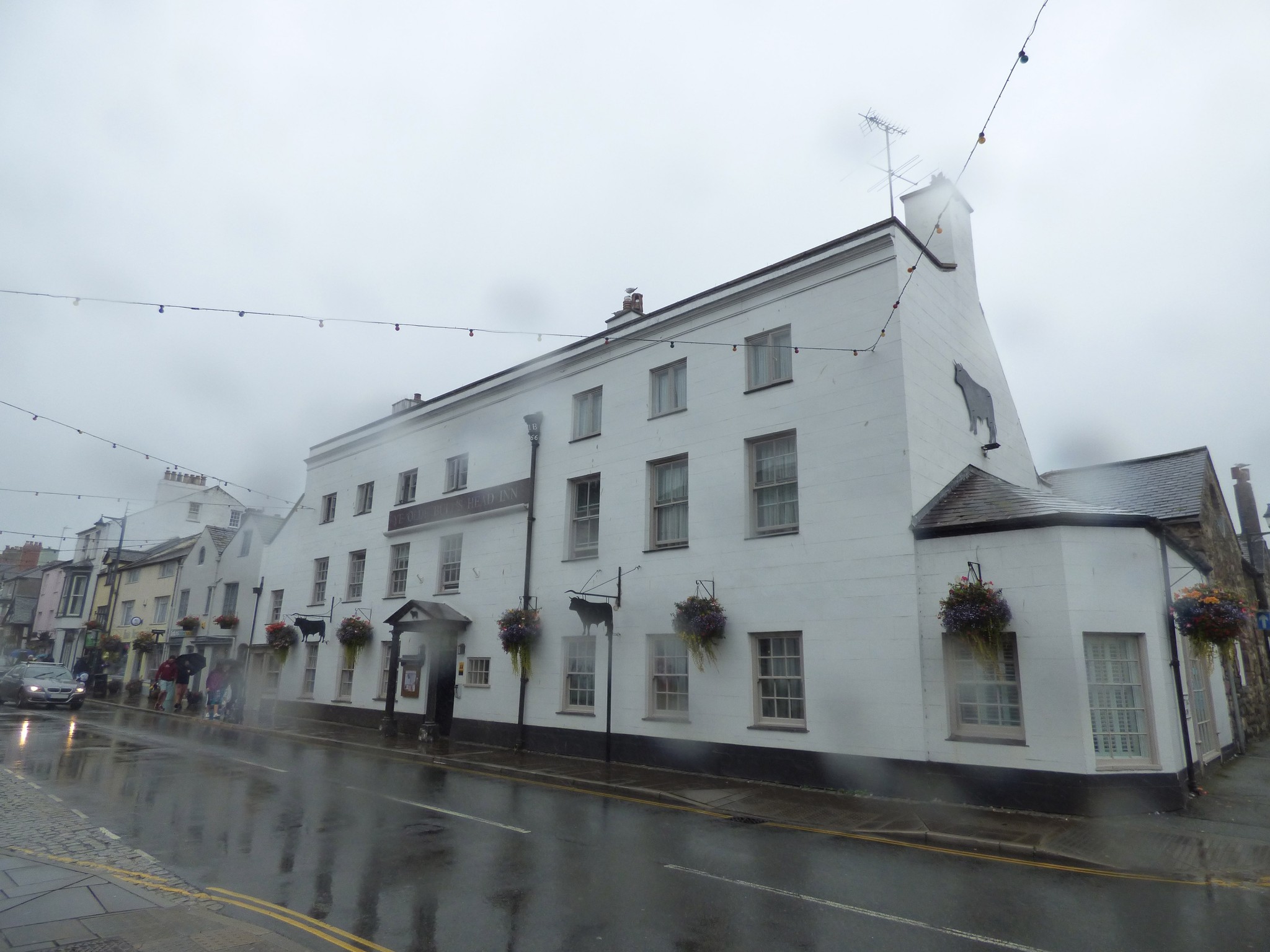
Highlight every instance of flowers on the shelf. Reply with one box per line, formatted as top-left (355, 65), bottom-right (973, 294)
top-left (496, 607), bottom-right (545, 680)
top-left (132, 632), bottom-right (155, 653)
top-left (82, 619), bottom-right (104, 630)
top-left (185, 689), bottom-right (204, 704)
top-left (937, 576), bottom-right (1014, 680)
top-left (213, 614), bottom-right (239, 625)
top-left (100, 634), bottom-right (122, 646)
top-left (1169, 581), bottom-right (1261, 678)
top-left (670, 596), bottom-right (728, 675)
top-left (176, 615), bottom-right (201, 627)
top-left (265, 621), bottom-right (300, 667)
top-left (336, 615), bottom-right (374, 671)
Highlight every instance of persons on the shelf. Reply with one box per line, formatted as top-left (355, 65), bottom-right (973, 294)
top-left (154, 655), bottom-right (178, 710)
top-left (26, 652), bottom-right (54, 662)
top-left (73, 655), bottom-right (87, 678)
top-left (205, 663), bottom-right (242, 719)
top-left (174, 659), bottom-right (193, 711)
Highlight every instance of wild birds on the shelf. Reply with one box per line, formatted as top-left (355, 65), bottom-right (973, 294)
top-left (624, 287), bottom-right (638, 296)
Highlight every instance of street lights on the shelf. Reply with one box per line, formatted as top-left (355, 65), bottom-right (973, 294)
top-left (93, 512), bottom-right (127, 674)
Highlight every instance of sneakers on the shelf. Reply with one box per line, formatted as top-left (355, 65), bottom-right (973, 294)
top-left (213, 713), bottom-right (220, 718)
top-left (206, 713), bottom-right (210, 717)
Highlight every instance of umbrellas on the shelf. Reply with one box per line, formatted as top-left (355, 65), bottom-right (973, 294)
top-left (11, 649), bottom-right (35, 657)
top-left (175, 653), bottom-right (206, 676)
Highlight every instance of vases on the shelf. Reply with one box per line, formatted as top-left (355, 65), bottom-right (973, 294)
top-left (221, 624), bottom-right (232, 629)
top-left (106, 646), bottom-right (118, 651)
top-left (187, 705), bottom-right (199, 712)
top-left (183, 626), bottom-right (194, 631)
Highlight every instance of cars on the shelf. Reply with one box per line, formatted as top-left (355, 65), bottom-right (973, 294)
top-left (0, 661), bottom-right (87, 711)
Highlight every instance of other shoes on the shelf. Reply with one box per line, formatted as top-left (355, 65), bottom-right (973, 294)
top-left (174, 703), bottom-right (181, 709)
top-left (154, 706), bottom-right (165, 711)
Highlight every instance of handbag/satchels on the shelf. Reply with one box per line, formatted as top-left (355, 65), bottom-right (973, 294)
top-left (222, 685), bottom-right (232, 703)
